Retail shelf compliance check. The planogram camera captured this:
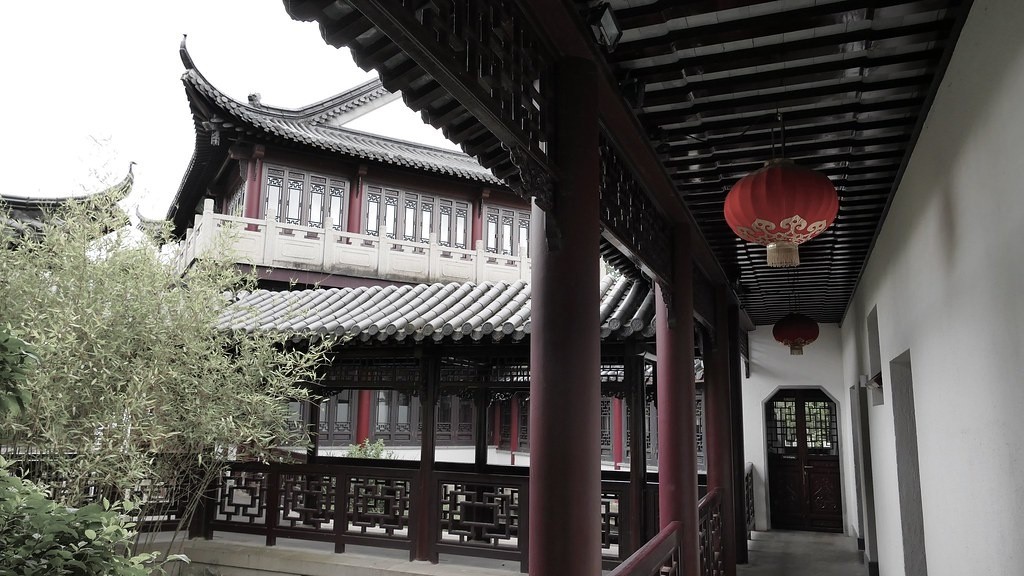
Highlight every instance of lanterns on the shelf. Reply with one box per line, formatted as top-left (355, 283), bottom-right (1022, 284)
top-left (723, 158), bottom-right (841, 268)
top-left (772, 313), bottom-right (820, 355)
